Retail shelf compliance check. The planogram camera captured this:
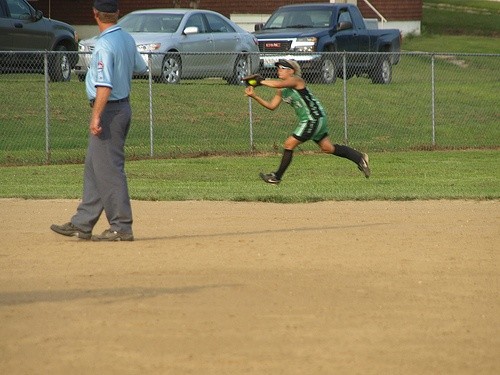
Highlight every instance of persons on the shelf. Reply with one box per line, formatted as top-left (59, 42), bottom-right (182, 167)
top-left (245, 59), bottom-right (371, 185)
top-left (50, 0), bottom-right (146, 242)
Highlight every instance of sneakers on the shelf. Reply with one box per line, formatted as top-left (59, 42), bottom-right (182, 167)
top-left (357, 153), bottom-right (370, 178)
top-left (50, 222), bottom-right (92, 240)
top-left (261, 171), bottom-right (281, 184)
top-left (90, 227), bottom-right (135, 241)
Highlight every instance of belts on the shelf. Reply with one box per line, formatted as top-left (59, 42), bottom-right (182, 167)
top-left (90, 98), bottom-right (129, 107)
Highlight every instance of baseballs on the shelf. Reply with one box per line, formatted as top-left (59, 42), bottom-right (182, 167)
top-left (248, 78), bottom-right (257, 85)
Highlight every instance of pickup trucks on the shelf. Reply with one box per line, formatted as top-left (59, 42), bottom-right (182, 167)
top-left (251, 3), bottom-right (403, 83)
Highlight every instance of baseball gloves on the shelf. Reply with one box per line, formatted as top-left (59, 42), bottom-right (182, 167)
top-left (244, 72), bottom-right (264, 87)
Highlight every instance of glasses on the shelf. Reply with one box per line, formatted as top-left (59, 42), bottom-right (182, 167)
top-left (275, 66), bottom-right (290, 70)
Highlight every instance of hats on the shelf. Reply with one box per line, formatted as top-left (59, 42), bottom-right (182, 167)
top-left (94, 0), bottom-right (118, 13)
top-left (274, 59), bottom-right (301, 77)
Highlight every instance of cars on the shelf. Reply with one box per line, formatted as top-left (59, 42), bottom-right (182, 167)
top-left (0, 0), bottom-right (80, 81)
top-left (74, 9), bottom-right (264, 84)
